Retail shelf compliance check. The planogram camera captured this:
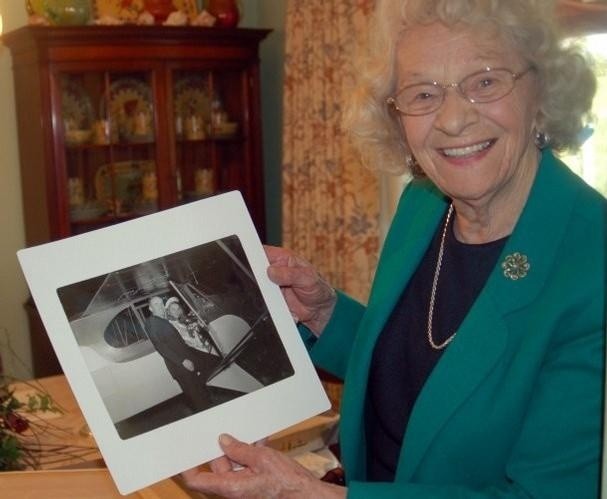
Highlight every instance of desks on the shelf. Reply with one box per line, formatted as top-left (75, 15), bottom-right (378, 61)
top-left (2, 369), bottom-right (346, 482)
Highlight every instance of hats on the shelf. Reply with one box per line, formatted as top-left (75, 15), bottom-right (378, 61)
top-left (165, 297), bottom-right (179, 309)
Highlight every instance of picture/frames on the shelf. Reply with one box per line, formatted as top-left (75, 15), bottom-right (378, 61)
top-left (14, 189), bottom-right (333, 497)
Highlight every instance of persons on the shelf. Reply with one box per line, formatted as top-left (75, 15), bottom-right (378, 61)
top-left (178, 1), bottom-right (606, 498)
top-left (145, 296), bottom-right (219, 413)
top-left (165, 297), bottom-right (210, 353)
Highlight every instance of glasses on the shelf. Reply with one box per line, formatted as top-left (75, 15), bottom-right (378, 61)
top-left (386, 66), bottom-right (532, 115)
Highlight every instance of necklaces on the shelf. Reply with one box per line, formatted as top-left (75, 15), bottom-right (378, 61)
top-left (428, 202), bottom-right (458, 348)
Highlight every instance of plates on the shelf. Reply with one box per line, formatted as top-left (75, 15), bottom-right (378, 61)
top-left (58, 80), bottom-right (237, 219)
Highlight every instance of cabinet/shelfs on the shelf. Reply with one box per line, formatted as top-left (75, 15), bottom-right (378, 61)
top-left (1, 24), bottom-right (277, 380)
